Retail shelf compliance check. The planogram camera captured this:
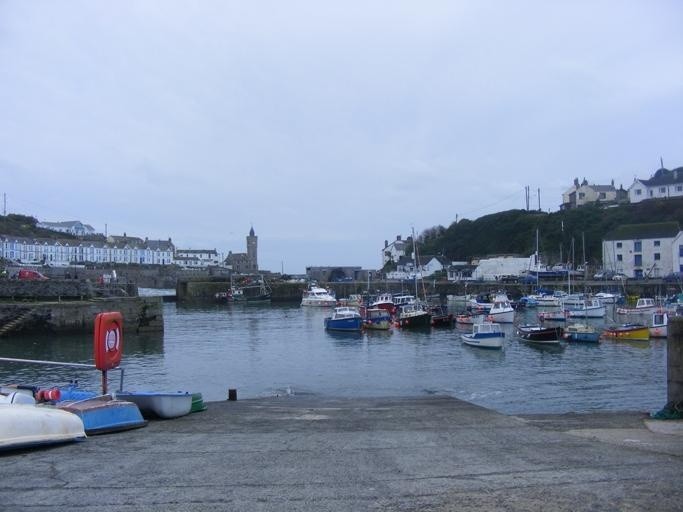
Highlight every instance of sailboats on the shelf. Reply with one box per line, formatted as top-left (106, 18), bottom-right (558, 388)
top-left (338, 225), bottom-right (626, 331)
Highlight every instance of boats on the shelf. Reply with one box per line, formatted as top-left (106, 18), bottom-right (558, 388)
top-left (460, 320), bottom-right (507, 348)
top-left (511, 318), bottom-right (562, 346)
top-left (635, 295), bottom-right (683, 315)
top-left (300, 279), bottom-right (337, 307)
top-left (0, 377), bottom-right (205, 455)
top-left (599, 321), bottom-right (650, 341)
top-left (561, 322), bottom-right (602, 344)
top-left (213, 271), bottom-right (273, 305)
top-left (640, 308), bottom-right (679, 338)
top-left (324, 305), bottom-right (364, 334)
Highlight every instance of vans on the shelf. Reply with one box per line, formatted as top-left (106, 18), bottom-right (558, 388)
top-left (18, 268), bottom-right (50, 282)
